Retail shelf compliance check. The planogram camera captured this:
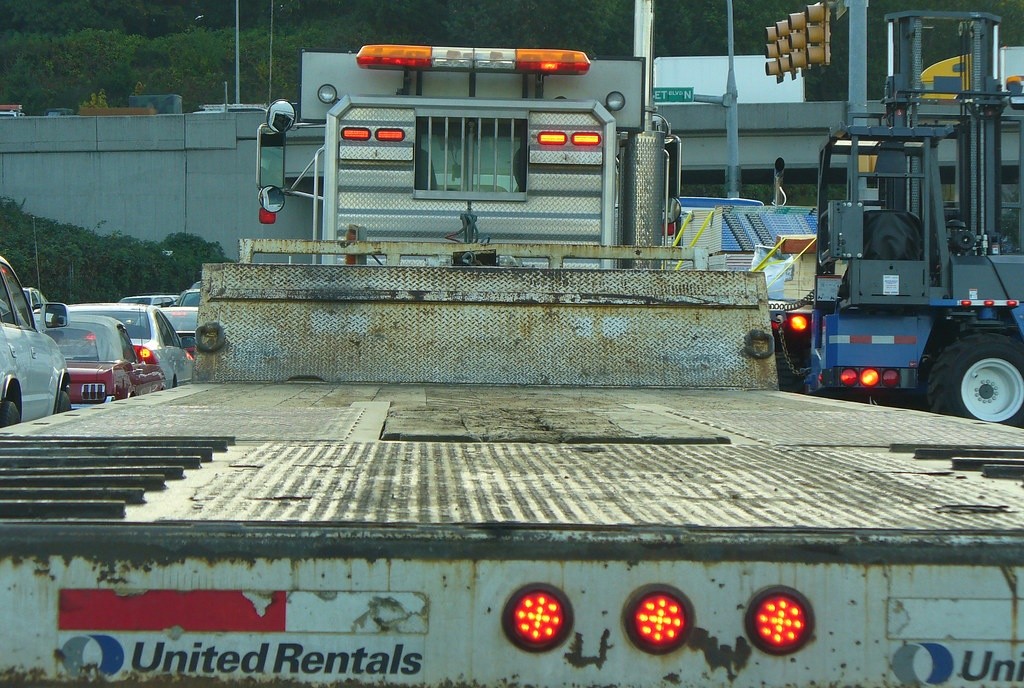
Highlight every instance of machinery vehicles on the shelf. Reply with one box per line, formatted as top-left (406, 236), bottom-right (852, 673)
top-left (807, 7), bottom-right (1024, 429)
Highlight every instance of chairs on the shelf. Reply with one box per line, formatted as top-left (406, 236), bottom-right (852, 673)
top-left (419, 150), bottom-right (438, 190)
top-left (514, 148), bottom-right (521, 187)
top-left (863, 211), bottom-right (924, 260)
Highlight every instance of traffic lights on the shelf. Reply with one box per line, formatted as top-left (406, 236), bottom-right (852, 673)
top-left (804, 2), bottom-right (832, 67)
top-left (764, 26), bottom-right (784, 85)
top-left (774, 19), bottom-right (798, 82)
top-left (788, 12), bottom-right (812, 71)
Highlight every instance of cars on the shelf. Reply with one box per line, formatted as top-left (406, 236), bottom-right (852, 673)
top-left (0, 104), bottom-right (26, 116)
top-left (0, 256), bottom-right (205, 429)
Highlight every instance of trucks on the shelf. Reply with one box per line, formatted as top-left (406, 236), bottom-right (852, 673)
top-left (674, 155), bottom-right (823, 354)
top-left (0, 42), bottom-right (1022, 686)
